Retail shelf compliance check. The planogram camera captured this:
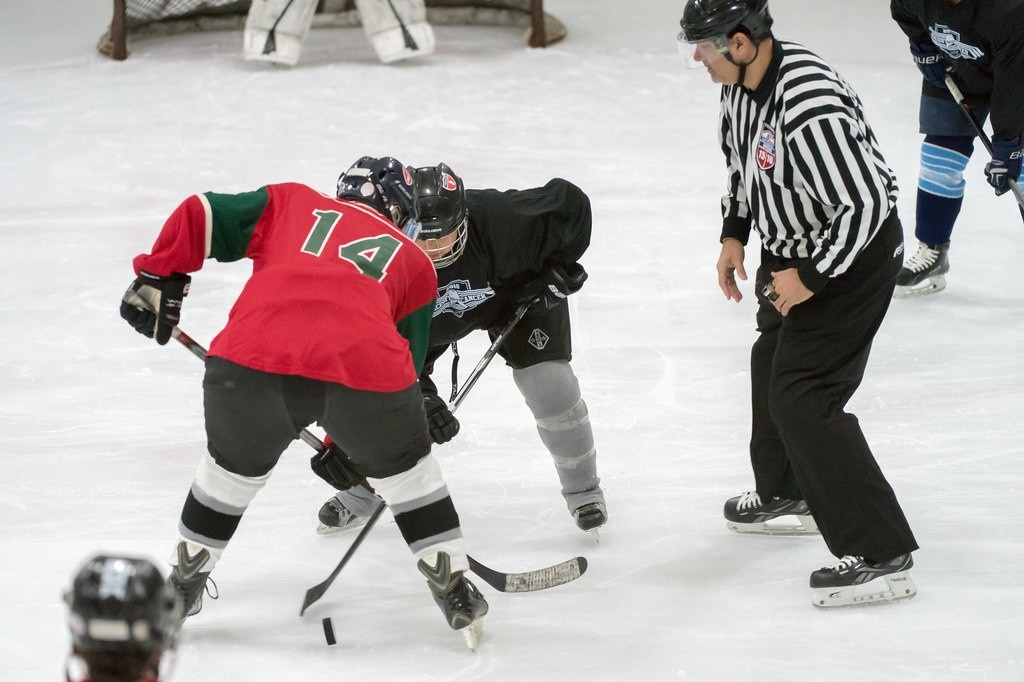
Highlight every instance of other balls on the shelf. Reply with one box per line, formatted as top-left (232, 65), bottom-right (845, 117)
top-left (322, 617), bottom-right (337, 647)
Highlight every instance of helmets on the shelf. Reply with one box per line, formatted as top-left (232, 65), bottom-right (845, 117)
top-left (678, 0), bottom-right (773, 55)
top-left (337, 155), bottom-right (419, 239)
top-left (404, 161), bottom-right (468, 266)
top-left (63, 553), bottom-right (175, 649)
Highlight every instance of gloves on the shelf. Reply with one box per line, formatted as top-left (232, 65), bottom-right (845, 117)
top-left (907, 40), bottom-right (955, 90)
top-left (418, 388), bottom-right (460, 445)
top-left (983, 139), bottom-right (1022, 196)
top-left (119, 270), bottom-right (192, 346)
top-left (310, 443), bottom-right (361, 492)
top-left (510, 263), bottom-right (588, 317)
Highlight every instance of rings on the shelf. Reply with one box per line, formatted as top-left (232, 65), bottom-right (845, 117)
top-left (770, 292), bottom-right (779, 302)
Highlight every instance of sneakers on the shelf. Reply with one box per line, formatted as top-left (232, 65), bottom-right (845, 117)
top-left (723, 493), bottom-right (822, 538)
top-left (570, 500), bottom-right (609, 546)
top-left (891, 239), bottom-right (951, 300)
top-left (314, 488), bottom-right (378, 535)
top-left (416, 551), bottom-right (490, 655)
top-left (166, 541), bottom-right (219, 634)
top-left (809, 546), bottom-right (919, 610)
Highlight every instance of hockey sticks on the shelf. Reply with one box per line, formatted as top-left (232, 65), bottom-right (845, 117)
top-left (944, 74), bottom-right (1024, 209)
top-left (300, 301), bottom-right (531, 616)
top-left (127, 293), bottom-right (589, 594)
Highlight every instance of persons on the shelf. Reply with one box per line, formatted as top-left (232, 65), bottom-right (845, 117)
top-left (892, 0), bottom-right (1024, 305)
top-left (316, 162), bottom-right (609, 543)
top-left (60, 554), bottom-right (170, 682)
top-left (243, 0), bottom-right (436, 66)
top-left (676, 0), bottom-right (920, 610)
top-left (121, 154), bottom-right (488, 652)
